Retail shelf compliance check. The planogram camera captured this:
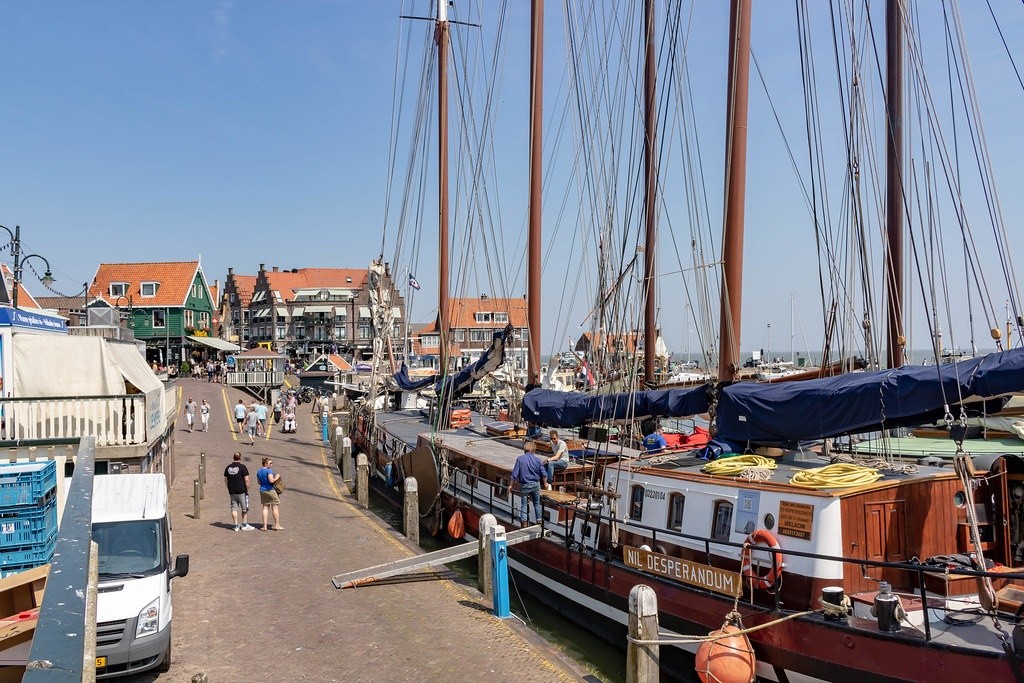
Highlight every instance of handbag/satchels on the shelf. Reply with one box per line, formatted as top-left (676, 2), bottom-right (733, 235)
top-left (272, 479), bottom-right (286, 494)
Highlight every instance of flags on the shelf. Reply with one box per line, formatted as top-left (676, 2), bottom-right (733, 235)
top-left (582, 363), bottom-right (595, 385)
top-left (409, 274), bottom-right (420, 290)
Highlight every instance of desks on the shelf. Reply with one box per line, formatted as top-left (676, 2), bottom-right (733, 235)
top-left (849, 592), bottom-right (945, 627)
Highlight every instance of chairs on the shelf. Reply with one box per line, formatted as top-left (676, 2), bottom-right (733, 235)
top-left (111, 522), bottom-right (150, 558)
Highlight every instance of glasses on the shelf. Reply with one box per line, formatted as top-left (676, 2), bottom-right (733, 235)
top-left (550, 438), bottom-right (557, 440)
top-left (266, 462), bottom-right (273, 465)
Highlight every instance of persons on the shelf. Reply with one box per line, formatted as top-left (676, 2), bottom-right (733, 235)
top-left (153, 360), bottom-right (176, 378)
top-left (639, 424), bottom-right (667, 456)
top-left (246, 359), bottom-right (270, 371)
top-left (193, 359), bottom-right (225, 384)
top-left (542, 429), bottom-right (569, 490)
top-left (273, 392), bottom-right (300, 433)
top-left (506, 441), bottom-right (548, 527)
top-left (224, 452), bottom-right (255, 532)
top-left (250, 399), bottom-right (269, 436)
top-left (184, 397), bottom-right (197, 432)
top-left (199, 400), bottom-right (210, 432)
top-left (243, 406), bottom-right (261, 446)
top-left (234, 400), bottom-right (246, 435)
top-left (256, 458), bottom-right (284, 531)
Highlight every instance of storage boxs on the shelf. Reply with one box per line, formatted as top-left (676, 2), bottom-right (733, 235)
top-left (0, 460), bottom-right (57, 653)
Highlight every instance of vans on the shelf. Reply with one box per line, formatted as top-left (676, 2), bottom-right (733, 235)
top-left (57, 469), bottom-right (189, 683)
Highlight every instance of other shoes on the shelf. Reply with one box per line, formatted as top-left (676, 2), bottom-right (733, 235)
top-left (251, 442), bottom-right (255, 446)
top-left (521, 521), bottom-right (528, 528)
top-left (547, 484), bottom-right (552, 490)
top-left (536, 519), bottom-right (542, 527)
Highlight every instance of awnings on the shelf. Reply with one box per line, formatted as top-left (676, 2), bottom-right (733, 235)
top-left (188, 336), bottom-right (248, 353)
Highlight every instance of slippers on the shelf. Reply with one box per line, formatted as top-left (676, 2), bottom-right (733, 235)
top-left (260, 528), bottom-right (267, 531)
top-left (274, 526), bottom-right (284, 531)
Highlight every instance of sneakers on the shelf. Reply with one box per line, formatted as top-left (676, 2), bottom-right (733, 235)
top-left (241, 523), bottom-right (255, 531)
top-left (231, 523), bottom-right (240, 531)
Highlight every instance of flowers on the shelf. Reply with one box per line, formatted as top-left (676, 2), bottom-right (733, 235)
top-left (186, 326), bottom-right (211, 332)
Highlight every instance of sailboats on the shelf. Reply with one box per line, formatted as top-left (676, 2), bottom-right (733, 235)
top-left (330, 0), bottom-right (1024, 683)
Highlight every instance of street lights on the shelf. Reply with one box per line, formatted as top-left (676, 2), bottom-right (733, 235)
top-left (0, 223), bottom-right (56, 311)
top-left (114, 294), bottom-right (148, 330)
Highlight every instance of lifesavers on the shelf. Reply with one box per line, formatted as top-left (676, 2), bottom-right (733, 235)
top-left (740, 531), bottom-right (785, 591)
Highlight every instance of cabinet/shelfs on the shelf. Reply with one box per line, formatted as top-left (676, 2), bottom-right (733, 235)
top-left (957, 520), bottom-right (1005, 561)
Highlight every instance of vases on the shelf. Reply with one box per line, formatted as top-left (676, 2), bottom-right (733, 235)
top-left (190, 332), bottom-right (207, 337)
